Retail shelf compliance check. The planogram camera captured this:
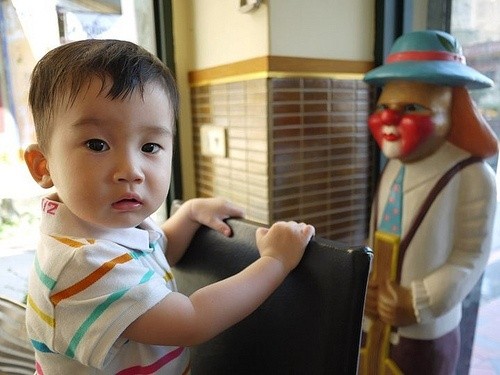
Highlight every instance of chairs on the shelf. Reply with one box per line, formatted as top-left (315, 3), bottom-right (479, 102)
top-left (165, 199), bottom-right (374, 375)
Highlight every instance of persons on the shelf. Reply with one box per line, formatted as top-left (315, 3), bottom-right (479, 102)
top-left (22, 39), bottom-right (316, 375)
top-left (354, 30), bottom-right (499, 375)
